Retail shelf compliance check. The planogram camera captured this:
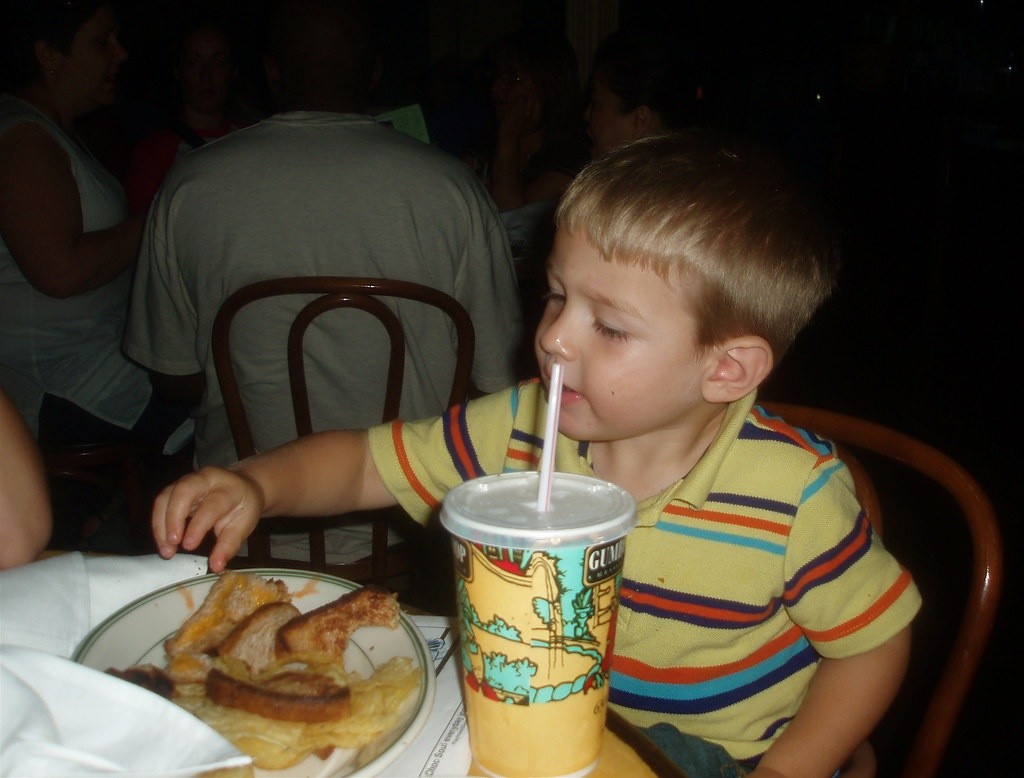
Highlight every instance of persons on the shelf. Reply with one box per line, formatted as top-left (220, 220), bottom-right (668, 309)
top-left (152, 132), bottom-right (922, 777)
top-left (0, 0), bottom-right (713, 567)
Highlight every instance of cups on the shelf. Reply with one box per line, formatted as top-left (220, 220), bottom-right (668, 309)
top-left (441, 471), bottom-right (638, 778)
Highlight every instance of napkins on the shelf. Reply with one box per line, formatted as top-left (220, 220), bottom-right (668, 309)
top-left (0, 550), bottom-right (252, 778)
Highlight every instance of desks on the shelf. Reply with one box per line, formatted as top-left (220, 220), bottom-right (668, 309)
top-left (0, 547), bottom-right (694, 778)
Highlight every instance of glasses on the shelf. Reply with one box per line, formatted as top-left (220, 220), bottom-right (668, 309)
top-left (496, 66), bottom-right (532, 83)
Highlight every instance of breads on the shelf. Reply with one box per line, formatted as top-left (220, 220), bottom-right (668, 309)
top-left (164, 572), bottom-right (399, 721)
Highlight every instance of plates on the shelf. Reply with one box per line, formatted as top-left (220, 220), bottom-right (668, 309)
top-left (73, 568), bottom-right (435, 778)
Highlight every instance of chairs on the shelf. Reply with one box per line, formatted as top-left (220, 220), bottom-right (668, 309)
top-left (211, 274), bottom-right (477, 619)
top-left (747, 398), bottom-right (1004, 778)
